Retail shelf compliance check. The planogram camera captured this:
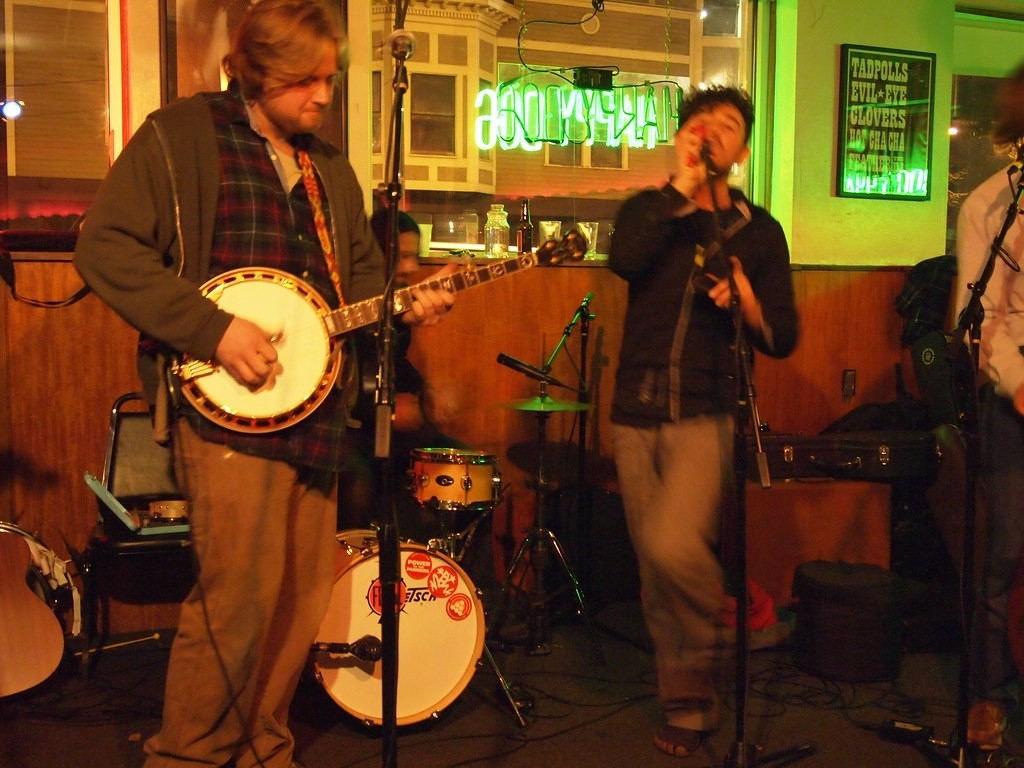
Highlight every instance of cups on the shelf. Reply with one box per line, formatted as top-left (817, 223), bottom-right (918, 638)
top-left (608, 223), bottom-right (615, 240)
top-left (417, 224), bottom-right (434, 258)
top-left (538, 220), bottom-right (561, 249)
top-left (576, 222), bottom-right (599, 256)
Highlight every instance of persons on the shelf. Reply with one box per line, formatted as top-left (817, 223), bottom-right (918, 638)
top-left (607, 82), bottom-right (799, 758)
top-left (342, 208), bottom-right (470, 529)
top-left (73, 0), bottom-right (460, 768)
top-left (955, 63), bottom-right (1024, 754)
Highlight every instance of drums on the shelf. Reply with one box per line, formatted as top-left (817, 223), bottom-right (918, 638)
top-left (305, 526), bottom-right (486, 730)
top-left (408, 447), bottom-right (503, 515)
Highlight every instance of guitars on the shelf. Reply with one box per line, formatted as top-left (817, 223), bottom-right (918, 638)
top-left (173, 225), bottom-right (591, 437)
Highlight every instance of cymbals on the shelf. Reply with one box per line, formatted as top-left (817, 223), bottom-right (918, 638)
top-left (489, 392), bottom-right (599, 416)
top-left (508, 438), bottom-right (618, 485)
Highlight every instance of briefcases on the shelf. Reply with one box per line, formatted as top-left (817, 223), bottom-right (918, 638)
top-left (752, 427), bottom-right (939, 482)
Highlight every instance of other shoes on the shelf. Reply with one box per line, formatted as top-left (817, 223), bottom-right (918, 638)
top-left (963, 701), bottom-right (1013, 754)
top-left (654, 724), bottom-right (704, 758)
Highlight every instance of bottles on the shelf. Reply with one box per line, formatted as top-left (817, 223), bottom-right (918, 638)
top-left (516, 198), bottom-right (534, 256)
top-left (483, 203), bottom-right (510, 257)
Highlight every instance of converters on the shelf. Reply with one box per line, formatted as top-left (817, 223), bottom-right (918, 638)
top-left (880, 719), bottom-right (935, 746)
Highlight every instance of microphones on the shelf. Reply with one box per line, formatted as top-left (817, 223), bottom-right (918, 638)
top-left (693, 125), bottom-right (712, 159)
top-left (388, 29), bottom-right (416, 59)
top-left (317, 635), bottom-right (382, 662)
top-left (1007, 145), bottom-right (1024, 176)
top-left (497, 353), bottom-right (562, 386)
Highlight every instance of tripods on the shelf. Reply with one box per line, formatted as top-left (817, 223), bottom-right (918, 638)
top-left (485, 411), bottom-right (608, 668)
